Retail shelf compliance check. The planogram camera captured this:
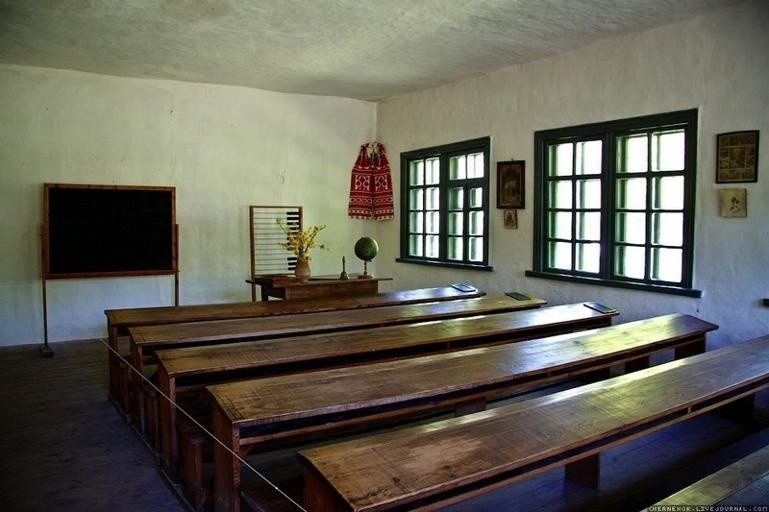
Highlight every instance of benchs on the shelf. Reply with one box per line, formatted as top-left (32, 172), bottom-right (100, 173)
top-left (104, 284), bottom-right (769, 512)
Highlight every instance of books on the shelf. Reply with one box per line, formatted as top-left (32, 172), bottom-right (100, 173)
top-left (451, 282), bottom-right (476, 293)
top-left (505, 292), bottom-right (531, 301)
top-left (584, 301), bottom-right (617, 314)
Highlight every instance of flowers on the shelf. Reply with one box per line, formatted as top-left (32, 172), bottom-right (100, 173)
top-left (274, 216), bottom-right (330, 260)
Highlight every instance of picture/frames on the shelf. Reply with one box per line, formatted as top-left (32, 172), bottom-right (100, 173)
top-left (716, 128), bottom-right (759, 184)
top-left (497, 160), bottom-right (525, 209)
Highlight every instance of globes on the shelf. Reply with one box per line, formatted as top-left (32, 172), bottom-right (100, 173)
top-left (354, 237), bottom-right (378, 279)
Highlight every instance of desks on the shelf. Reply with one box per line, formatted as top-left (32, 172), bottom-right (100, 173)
top-left (246, 271), bottom-right (391, 300)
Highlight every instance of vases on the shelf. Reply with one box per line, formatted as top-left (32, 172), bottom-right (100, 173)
top-left (295, 259), bottom-right (311, 282)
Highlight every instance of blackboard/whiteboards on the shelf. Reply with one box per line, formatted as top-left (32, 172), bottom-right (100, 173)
top-left (43, 183), bottom-right (178, 279)
